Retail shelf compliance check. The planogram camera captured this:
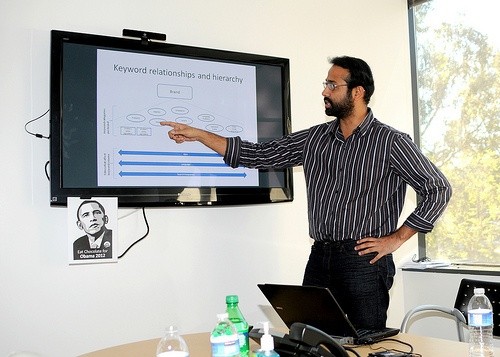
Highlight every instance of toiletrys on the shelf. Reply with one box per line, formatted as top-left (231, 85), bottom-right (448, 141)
top-left (252, 320), bottom-right (281, 356)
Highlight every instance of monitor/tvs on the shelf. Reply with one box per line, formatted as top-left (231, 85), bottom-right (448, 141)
top-left (49, 29), bottom-right (297, 208)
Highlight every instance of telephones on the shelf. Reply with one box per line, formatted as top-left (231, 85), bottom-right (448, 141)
top-left (250, 321), bottom-right (351, 357)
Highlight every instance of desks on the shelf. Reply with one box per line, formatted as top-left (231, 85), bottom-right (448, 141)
top-left (77, 329), bottom-right (500, 357)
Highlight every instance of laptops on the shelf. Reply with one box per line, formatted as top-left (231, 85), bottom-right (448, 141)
top-left (257, 283), bottom-right (400, 346)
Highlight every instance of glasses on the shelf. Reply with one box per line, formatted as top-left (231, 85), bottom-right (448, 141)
top-left (322, 82), bottom-right (348, 92)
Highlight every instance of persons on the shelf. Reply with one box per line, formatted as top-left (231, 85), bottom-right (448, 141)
top-left (160, 56), bottom-right (453, 331)
top-left (72, 200), bottom-right (113, 260)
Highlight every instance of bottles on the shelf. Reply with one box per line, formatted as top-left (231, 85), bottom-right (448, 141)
top-left (156, 325), bottom-right (190, 357)
top-left (225, 294), bottom-right (250, 357)
top-left (209, 312), bottom-right (241, 357)
top-left (467, 287), bottom-right (495, 357)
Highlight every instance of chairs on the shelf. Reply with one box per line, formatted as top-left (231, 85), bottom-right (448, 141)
top-left (400, 305), bottom-right (470, 343)
